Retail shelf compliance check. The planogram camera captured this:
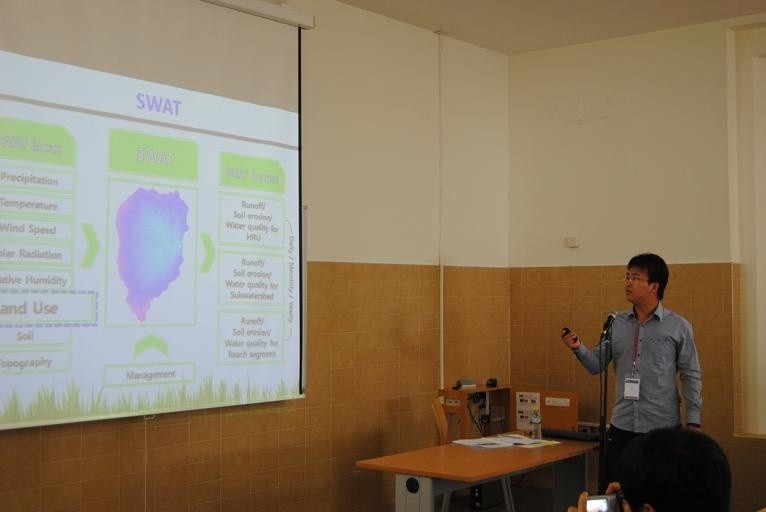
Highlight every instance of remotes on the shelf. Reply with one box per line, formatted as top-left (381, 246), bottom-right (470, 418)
top-left (561, 327), bottom-right (577, 345)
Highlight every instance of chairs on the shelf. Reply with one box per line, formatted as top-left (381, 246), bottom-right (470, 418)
top-left (432, 399), bottom-right (515, 511)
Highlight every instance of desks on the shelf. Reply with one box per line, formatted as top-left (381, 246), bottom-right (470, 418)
top-left (356, 427), bottom-right (600, 512)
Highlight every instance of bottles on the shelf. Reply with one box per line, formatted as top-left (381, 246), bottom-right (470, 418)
top-left (531, 408), bottom-right (542, 443)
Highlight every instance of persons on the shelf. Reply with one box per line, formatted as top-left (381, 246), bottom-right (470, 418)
top-left (560, 252), bottom-right (703, 489)
top-left (567, 427), bottom-right (732, 512)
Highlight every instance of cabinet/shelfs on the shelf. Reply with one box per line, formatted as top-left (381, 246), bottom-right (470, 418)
top-left (439, 384), bottom-right (578, 446)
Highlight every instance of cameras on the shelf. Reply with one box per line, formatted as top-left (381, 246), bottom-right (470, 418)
top-left (586, 494), bottom-right (623, 512)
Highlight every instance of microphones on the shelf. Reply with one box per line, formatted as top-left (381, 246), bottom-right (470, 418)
top-left (601, 309), bottom-right (617, 341)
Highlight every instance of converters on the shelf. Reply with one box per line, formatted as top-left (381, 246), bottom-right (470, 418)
top-left (486, 378), bottom-right (497, 386)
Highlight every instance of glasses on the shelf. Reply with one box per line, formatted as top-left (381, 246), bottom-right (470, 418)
top-left (623, 274), bottom-right (654, 283)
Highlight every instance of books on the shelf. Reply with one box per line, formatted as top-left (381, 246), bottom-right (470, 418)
top-left (452, 436), bottom-right (541, 449)
